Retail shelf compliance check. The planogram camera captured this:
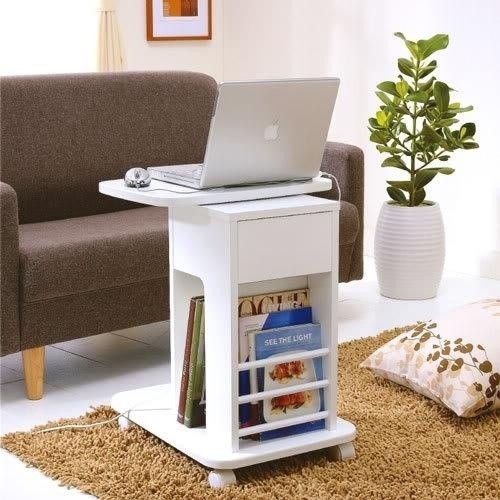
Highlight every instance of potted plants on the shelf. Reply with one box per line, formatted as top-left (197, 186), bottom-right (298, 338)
top-left (366, 32), bottom-right (480, 300)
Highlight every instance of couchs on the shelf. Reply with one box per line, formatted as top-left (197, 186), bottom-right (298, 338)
top-left (1, 72), bottom-right (364, 400)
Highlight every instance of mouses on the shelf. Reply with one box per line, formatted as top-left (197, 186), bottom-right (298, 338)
top-left (124, 167), bottom-right (152, 188)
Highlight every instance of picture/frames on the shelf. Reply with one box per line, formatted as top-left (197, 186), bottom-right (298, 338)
top-left (147, 0), bottom-right (212, 41)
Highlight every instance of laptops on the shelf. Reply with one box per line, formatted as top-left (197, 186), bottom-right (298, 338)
top-left (147, 76), bottom-right (340, 189)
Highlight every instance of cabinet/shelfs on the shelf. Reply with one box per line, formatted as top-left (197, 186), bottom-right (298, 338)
top-left (112, 193), bottom-right (358, 488)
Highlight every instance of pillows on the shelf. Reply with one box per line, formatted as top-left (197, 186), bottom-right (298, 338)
top-left (358, 296), bottom-right (499, 417)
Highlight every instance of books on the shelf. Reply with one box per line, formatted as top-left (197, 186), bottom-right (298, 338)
top-left (182, 292), bottom-right (206, 428)
top-left (247, 320), bottom-right (328, 441)
top-left (236, 304), bottom-right (318, 442)
top-left (238, 284), bottom-right (311, 314)
top-left (178, 297), bottom-right (207, 425)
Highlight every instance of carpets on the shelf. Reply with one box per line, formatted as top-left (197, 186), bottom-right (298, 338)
top-left (0, 323), bottom-right (499, 500)
